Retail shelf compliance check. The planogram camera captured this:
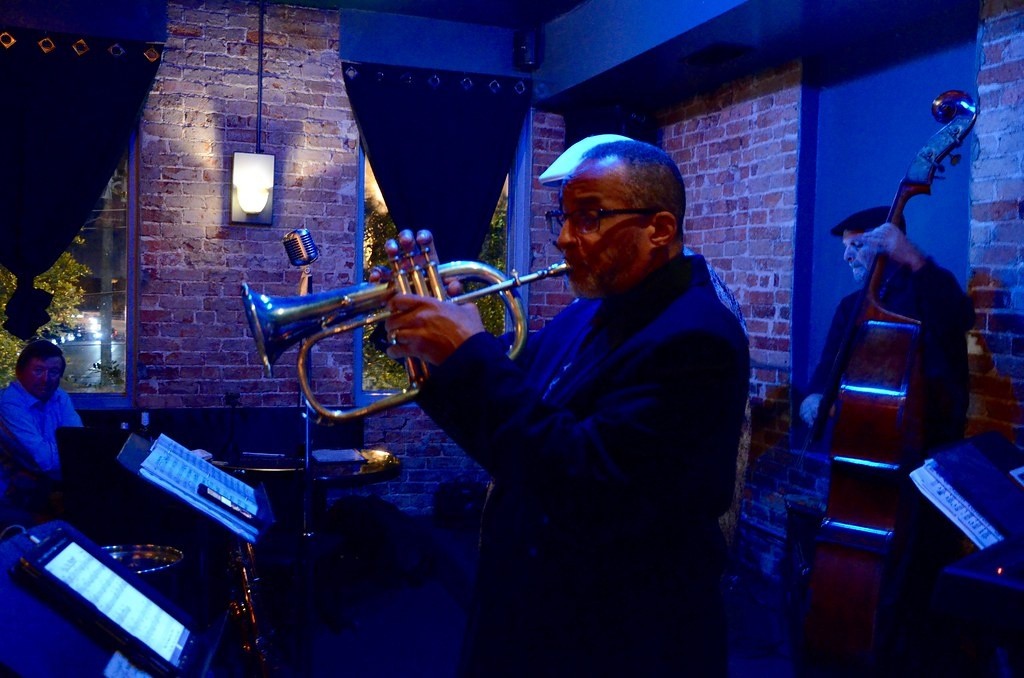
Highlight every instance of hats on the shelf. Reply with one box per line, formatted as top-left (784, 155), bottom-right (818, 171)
top-left (830, 205), bottom-right (907, 237)
top-left (536, 134), bottom-right (639, 184)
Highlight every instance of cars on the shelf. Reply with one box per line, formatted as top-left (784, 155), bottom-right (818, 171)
top-left (61, 314), bottom-right (120, 342)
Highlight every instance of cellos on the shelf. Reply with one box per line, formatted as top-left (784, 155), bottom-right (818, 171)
top-left (786, 86), bottom-right (982, 678)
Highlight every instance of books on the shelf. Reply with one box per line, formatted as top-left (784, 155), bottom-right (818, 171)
top-left (138, 433), bottom-right (258, 542)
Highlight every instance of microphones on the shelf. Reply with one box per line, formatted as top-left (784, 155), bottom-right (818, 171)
top-left (284, 227), bottom-right (318, 265)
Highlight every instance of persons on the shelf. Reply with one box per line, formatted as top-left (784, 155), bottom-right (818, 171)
top-left (369, 133), bottom-right (753, 678)
top-left (0, 339), bottom-right (84, 530)
top-left (798, 206), bottom-right (974, 576)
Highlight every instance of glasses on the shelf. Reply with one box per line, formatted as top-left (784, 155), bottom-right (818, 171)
top-left (545, 207), bottom-right (667, 234)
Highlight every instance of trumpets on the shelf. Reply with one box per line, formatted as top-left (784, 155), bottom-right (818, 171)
top-left (237, 246), bottom-right (583, 423)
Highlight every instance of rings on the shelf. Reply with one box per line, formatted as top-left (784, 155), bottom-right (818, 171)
top-left (391, 329), bottom-right (398, 343)
